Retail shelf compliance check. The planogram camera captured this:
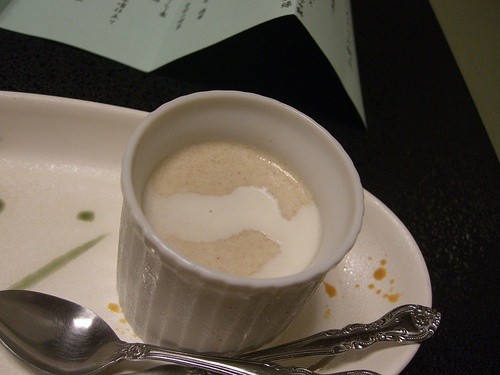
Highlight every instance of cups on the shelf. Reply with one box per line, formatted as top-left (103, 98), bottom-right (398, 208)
top-left (115, 90), bottom-right (366, 355)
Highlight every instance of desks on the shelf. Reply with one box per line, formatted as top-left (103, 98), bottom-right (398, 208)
top-left (0, 1), bottom-right (500, 375)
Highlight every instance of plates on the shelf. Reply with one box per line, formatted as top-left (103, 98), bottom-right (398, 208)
top-left (0, 91), bottom-right (432, 375)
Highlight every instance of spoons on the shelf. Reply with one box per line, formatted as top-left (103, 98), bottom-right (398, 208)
top-left (1, 287), bottom-right (383, 375)
